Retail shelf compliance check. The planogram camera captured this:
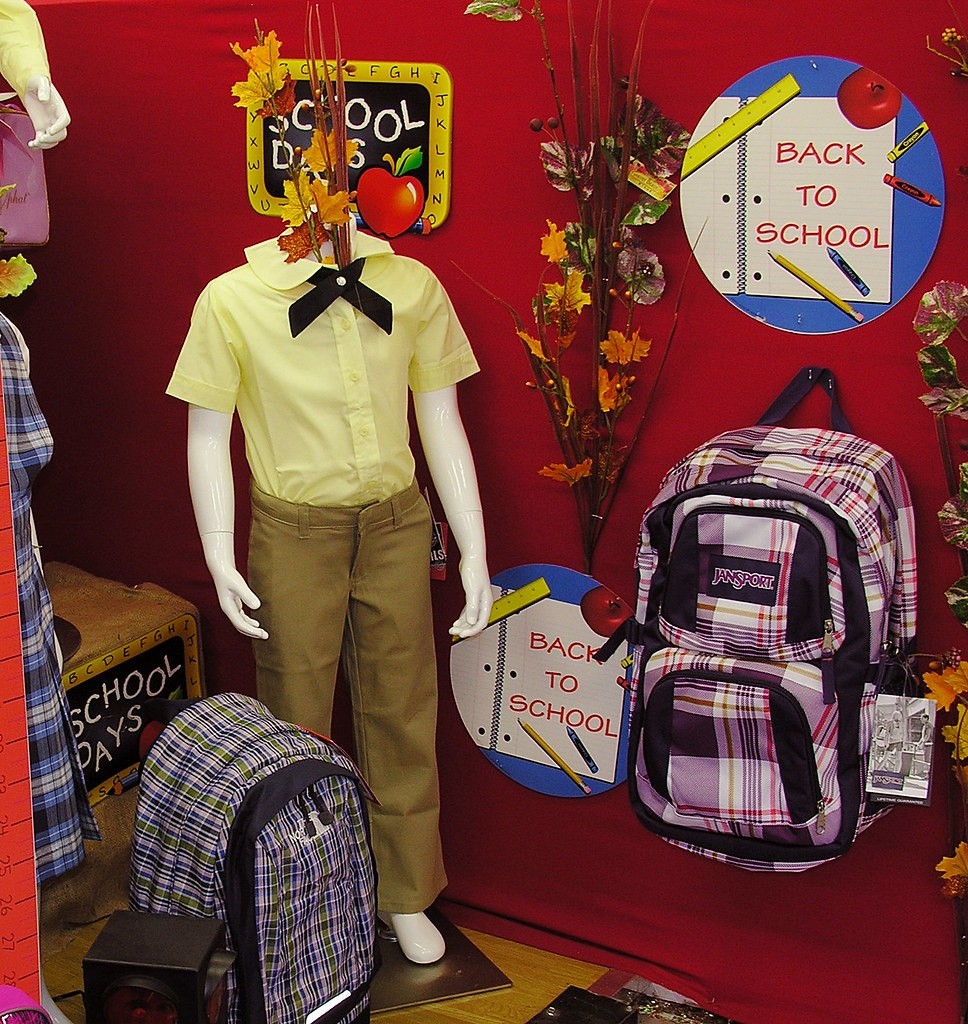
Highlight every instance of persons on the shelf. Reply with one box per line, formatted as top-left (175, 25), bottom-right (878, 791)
top-left (0, 0), bottom-right (102, 884)
top-left (166, 207), bottom-right (492, 965)
top-left (874, 707), bottom-right (933, 786)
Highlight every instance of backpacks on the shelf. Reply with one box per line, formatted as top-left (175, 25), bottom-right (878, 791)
top-left (129, 692), bottom-right (378, 1024)
top-left (593, 363), bottom-right (917, 872)
